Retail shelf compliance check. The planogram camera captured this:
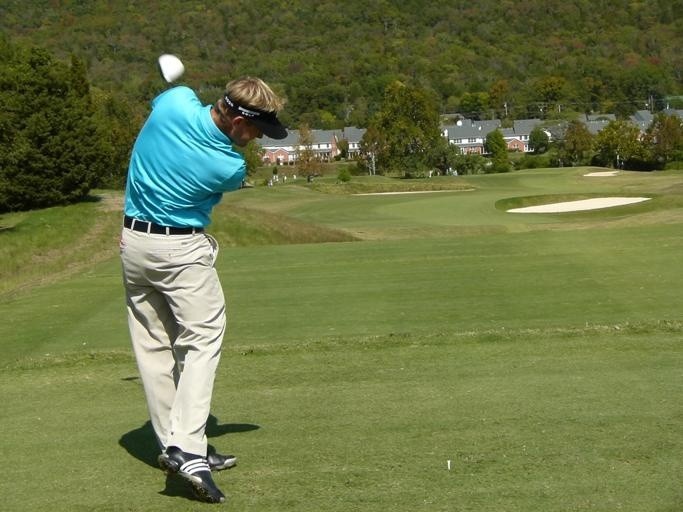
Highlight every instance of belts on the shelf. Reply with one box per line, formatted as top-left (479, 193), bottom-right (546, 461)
top-left (122, 215), bottom-right (203, 235)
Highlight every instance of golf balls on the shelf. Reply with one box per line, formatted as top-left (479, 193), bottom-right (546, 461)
top-left (456, 120), bottom-right (462, 127)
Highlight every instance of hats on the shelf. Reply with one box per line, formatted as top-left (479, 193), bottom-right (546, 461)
top-left (224, 92), bottom-right (288, 140)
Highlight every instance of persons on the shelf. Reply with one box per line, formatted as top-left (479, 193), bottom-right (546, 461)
top-left (117, 74), bottom-right (289, 504)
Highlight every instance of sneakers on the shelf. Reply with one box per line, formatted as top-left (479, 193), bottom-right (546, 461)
top-left (159, 446), bottom-right (227, 505)
top-left (157, 443), bottom-right (237, 470)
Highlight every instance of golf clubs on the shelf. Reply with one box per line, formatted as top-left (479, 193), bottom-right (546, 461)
top-left (159, 50), bottom-right (185, 90)
